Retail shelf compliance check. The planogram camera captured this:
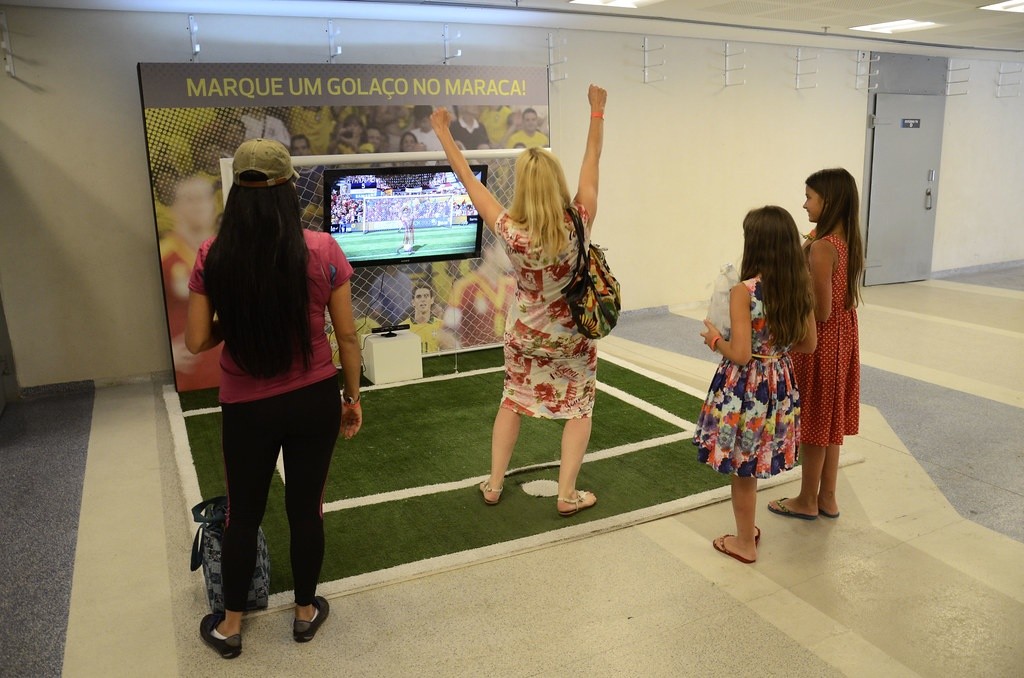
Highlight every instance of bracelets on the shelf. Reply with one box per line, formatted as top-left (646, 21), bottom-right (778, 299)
top-left (342, 401), bottom-right (360, 410)
top-left (710, 334), bottom-right (722, 352)
top-left (589, 111), bottom-right (605, 121)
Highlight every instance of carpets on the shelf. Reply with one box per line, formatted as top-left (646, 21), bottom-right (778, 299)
top-left (160, 347), bottom-right (871, 624)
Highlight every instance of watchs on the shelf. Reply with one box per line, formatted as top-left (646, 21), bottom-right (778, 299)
top-left (342, 388), bottom-right (361, 405)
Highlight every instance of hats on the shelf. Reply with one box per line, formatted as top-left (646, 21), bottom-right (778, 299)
top-left (233, 138), bottom-right (300, 188)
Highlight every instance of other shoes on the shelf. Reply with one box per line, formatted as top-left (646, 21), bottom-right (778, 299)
top-left (292, 595), bottom-right (329, 642)
top-left (200, 613), bottom-right (241, 659)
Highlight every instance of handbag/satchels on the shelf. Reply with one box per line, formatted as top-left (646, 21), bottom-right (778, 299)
top-left (561, 205), bottom-right (621, 340)
top-left (189, 494), bottom-right (271, 613)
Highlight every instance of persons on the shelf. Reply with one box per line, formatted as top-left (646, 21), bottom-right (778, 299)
top-left (700, 205), bottom-right (819, 565)
top-left (764, 166), bottom-right (863, 518)
top-left (429, 83), bottom-right (607, 519)
top-left (185, 134), bottom-right (368, 658)
top-left (150, 87), bottom-right (550, 412)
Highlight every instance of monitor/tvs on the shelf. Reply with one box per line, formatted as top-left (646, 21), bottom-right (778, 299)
top-left (323, 163), bottom-right (487, 267)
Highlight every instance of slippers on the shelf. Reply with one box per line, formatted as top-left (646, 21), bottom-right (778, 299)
top-left (754, 526), bottom-right (760, 548)
top-left (768, 497), bottom-right (816, 520)
top-left (819, 508), bottom-right (839, 518)
top-left (713, 534), bottom-right (755, 563)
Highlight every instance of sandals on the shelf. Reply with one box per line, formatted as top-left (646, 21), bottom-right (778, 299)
top-left (482, 479), bottom-right (503, 505)
top-left (557, 489), bottom-right (595, 516)
